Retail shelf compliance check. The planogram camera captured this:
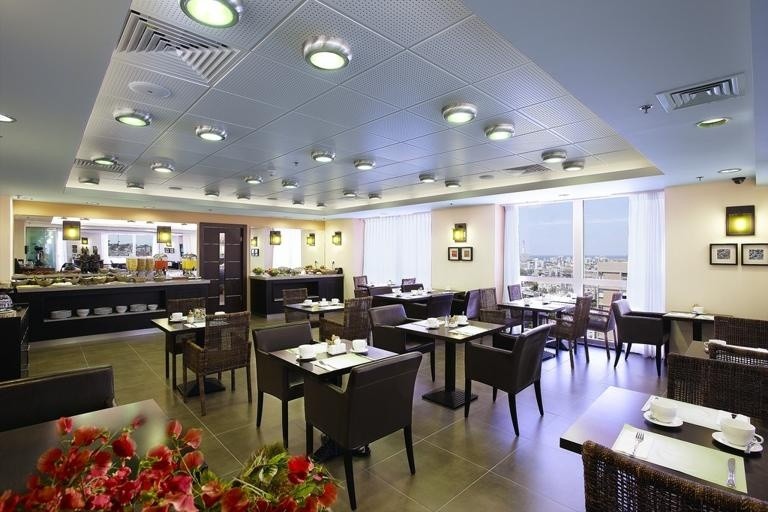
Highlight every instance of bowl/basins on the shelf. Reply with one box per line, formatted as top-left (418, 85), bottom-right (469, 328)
top-left (36, 278), bottom-right (53, 287)
top-left (116, 305), bottom-right (128, 314)
top-left (93, 277), bottom-right (115, 284)
top-left (75, 308), bottom-right (89, 317)
top-left (148, 303), bottom-right (158, 311)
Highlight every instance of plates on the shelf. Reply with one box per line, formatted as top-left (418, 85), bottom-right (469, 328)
top-left (168, 320), bottom-right (187, 322)
top-left (456, 322), bottom-right (469, 326)
top-left (50, 309), bottom-right (71, 319)
top-left (425, 326), bottom-right (438, 329)
top-left (643, 411), bottom-right (682, 429)
top-left (710, 431), bottom-right (763, 454)
top-left (295, 354), bottom-right (315, 360)
top-left (129, 304), bottom-right (146, 312)
top-left (349, 350), bottom-right (368, 353)
top-left (94, 307), bottom-right (112, 315)
top-left (300, 303), bottom-right (340, 307)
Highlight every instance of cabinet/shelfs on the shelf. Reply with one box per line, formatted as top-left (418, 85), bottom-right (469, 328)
top-left (14, 278), bottom-right (209, 341)
top-left (249, 273), bottom-right (345, 319)
top-left (0, 303), bottom-right (31, 380)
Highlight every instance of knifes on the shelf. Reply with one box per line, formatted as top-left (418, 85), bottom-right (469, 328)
top-left (726, 457), bottom-right (735, 489)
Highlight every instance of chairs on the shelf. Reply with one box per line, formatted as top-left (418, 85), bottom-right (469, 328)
top-left (150, 276), bottom-right (768, 511)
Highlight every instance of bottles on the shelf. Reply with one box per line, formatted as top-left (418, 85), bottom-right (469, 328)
top-left (186, 310), bottom-right (195, 325)
top-left (443, 313), bottom-right (448, 327)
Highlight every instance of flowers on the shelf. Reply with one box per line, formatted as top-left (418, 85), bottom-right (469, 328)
top-left (1, 413), bottom-right (355, 510)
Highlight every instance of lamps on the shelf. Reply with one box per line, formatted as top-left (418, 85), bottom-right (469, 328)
top-left (269, 230), bottom-right (282, 246)
top-left (250, 236), bottom-right (258, 248)
top-left (38, 0), bottom-right (608, 209)
top-left (61, 220), bottom-right (83, 243)
top-left (725, 205), bottom-right (756, 236)
top-left (155, 224), bottom-right (173, 245)
top-left (331, 231), bottom-right (344, 246)
top-left (305, 232), bottom-right (316, 247)
top-left (452, 222), bottom-right (467, 242)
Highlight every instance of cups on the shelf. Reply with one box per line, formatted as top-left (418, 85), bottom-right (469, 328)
top-left (650, 397), bottom-right (675, 423)
top-left (720, 417), bottom-right (764, 448)
top-left (169, 312), bottom-right (183, 320)
top-left (703, 338), bottom-right (726, 348)
top-left (303, 298), bottom-right (337, 304)
top-left (393, 287), bottom-right (424, 297)
top-left (692, 307), bottom-right (702, 313)
top-left (352, 339), bottom-right (366, 350)
top-left (297, 344), bottom-right (314, 356)
top-left (455, 315), bottom-right (466, 322)
top-left (426, 318), bottom-right (436, 326)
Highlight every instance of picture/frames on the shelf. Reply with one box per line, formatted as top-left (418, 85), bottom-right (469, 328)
top-left (447, 246), bottom-right (459, 260)
top-left (740, 243), bottom-right (768, 265)
top-left (710, 242), bottom-right (738, 265)
top-left (460, 246), bottom-right (473, 260)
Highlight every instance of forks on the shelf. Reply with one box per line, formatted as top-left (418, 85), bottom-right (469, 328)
top-left (627, 431), bottom-right (645, 457)
top-left (319, 360), bottom-right (340, 370)
